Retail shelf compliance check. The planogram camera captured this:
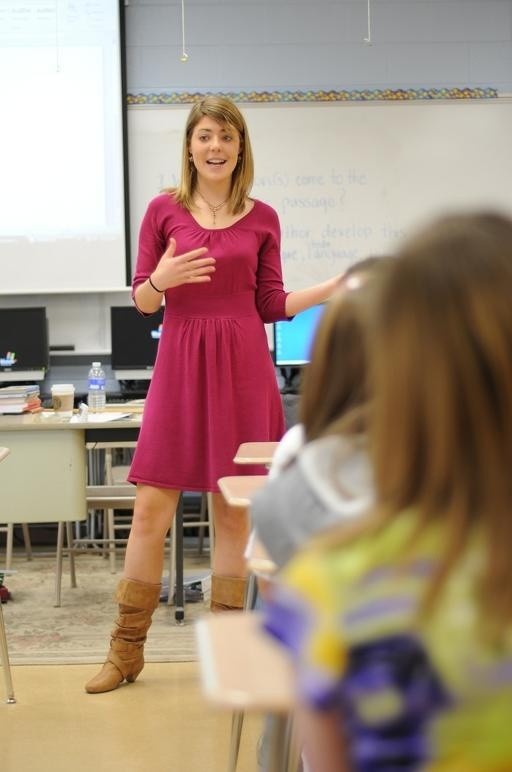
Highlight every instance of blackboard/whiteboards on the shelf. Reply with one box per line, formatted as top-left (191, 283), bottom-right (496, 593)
top-left (127, 91), bottom-right (509, 305)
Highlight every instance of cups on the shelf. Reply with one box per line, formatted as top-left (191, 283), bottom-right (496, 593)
top-left (51, 384), bottom-right (76, 418)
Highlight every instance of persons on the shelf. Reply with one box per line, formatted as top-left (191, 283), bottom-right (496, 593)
top-left (246, 260), bottom-right (398, 585)
top-left (260, 213), bottom-right (512, 772)
top-left (86, 96), bottom-right (352, 694)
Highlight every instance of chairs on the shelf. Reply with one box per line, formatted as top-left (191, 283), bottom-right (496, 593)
top-left (56, 442), bottom-right (177, 607)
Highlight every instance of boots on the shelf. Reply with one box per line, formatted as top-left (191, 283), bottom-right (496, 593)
top-left (85, 578), bottom-right (162, 693)
top-left (210, 575), bottom-right (246, 615)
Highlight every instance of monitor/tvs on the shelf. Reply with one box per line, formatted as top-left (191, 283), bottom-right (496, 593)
top-left (273, 304), bottom-right (338, 394)
top-left (0, 307), bottom-right (49, 372)
top-left (110, 305), bottom-right (167, 370)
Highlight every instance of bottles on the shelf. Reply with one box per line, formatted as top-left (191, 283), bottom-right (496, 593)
top-left (88, 361), bottom-right (106, 413)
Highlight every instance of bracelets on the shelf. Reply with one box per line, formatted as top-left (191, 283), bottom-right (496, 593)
top-left (148, 275), bottom-right (167, 293)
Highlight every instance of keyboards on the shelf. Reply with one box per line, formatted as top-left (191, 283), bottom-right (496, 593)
top-left (74, 390), bottom-right (148, 409)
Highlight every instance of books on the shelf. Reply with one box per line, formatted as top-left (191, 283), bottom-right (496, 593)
top-left (0, 385), bottom-right (40, 415)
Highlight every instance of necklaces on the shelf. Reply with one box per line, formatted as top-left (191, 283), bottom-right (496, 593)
top-left (192, 184), bottom-right (232, 222)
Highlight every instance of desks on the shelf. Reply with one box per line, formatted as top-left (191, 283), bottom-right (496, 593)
top-left (2, 409), bottom-right (140, 523)
top-left (199, 614), bottom-right (296, 772)
